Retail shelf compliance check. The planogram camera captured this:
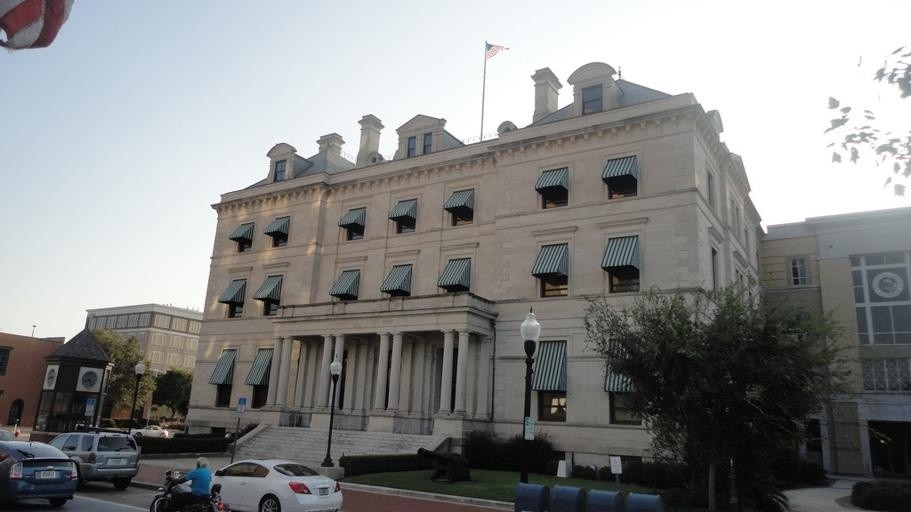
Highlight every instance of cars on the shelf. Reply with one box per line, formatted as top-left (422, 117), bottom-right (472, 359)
top-left (209, 459), bottom-right (345, 512)
top-left (0, 439), bottom-right (80, 508)
top-left (131, 424), bottom-right (171, 438)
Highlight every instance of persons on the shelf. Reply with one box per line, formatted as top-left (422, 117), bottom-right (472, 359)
top-left (160, 425), bottom-right (170, 438)
top-left (166, 456), bottom-right (213, 512)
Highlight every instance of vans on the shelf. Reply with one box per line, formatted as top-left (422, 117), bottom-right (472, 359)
top-left (47, 432), bottom-right (142, 491)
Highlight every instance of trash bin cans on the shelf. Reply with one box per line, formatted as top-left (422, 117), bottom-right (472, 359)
top-left (209, 426), bottom-right (226, 451)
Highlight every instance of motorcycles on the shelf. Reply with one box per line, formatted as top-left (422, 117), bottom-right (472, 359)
top-left (150, 471), bottom-right (224, 512)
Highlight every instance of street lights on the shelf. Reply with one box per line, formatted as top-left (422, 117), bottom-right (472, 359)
top-left (319, 355), bottom-right (344, 468)
top-left (127, 360), bottom-right (147, 434)
top-left (520, 305), bottom-right (541, 482)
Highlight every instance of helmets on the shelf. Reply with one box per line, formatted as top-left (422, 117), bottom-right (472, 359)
top-left (197, 457), bottom-right (207, 468)
